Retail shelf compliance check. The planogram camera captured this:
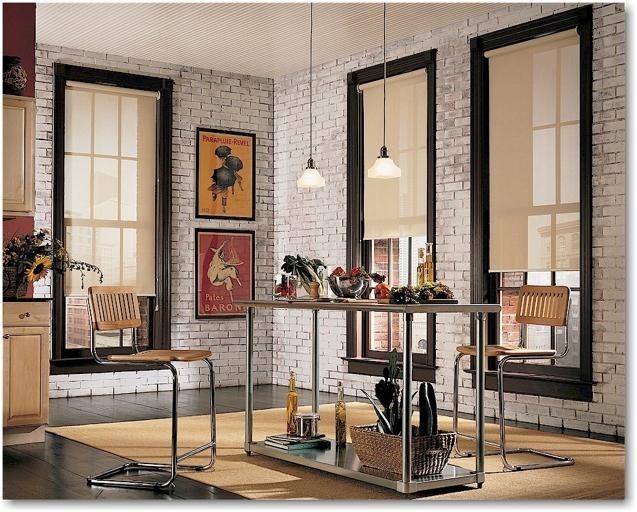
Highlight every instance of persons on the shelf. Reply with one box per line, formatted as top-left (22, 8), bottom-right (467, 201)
top-left (208, 157), bottom-right (244, 213)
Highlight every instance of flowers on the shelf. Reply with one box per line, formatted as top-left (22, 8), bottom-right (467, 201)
top-left (3, 227), bottom-right (103, 290)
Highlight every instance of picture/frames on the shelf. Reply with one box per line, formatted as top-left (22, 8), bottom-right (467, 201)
top-left (193, 227), bottom-right (255, 320)
top-left (195, 127), bottom-right (256, 221)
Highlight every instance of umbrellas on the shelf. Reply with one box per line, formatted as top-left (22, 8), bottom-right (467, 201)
top-left (211, 145), bottom-right (244, 187)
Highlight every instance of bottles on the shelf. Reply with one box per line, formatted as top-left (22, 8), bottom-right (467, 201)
top-left (335, 385), bottom-right (346, 446)
top-left (293, 413), bottom-right (321, 438)
top-left (287, 370), bottom-right (298, 437)
top-left (424, 243), bottom-right (435, 284)
top-left (417, 247), bottom-right (425, 286)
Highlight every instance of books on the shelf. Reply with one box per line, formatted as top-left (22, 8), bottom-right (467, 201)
top-left (265, 434), bottom-right (331, 450)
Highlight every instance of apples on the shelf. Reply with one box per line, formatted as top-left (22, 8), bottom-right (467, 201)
top-left (375, 283), bottom-right (390, 299)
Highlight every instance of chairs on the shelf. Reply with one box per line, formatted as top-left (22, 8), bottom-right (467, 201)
top-left (452, 285), bottom-right (574, 472)
top-left (85, 284), bottom-right (217, 488)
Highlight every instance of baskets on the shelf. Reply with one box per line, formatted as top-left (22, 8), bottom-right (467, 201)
top-left (349, 423), bottom-right (457, 476)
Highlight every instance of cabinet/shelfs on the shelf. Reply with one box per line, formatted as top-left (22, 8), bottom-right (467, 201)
top-left (2, 98), bottom-right (36, 218)
top-left (3, 301), bottom-right (51, 427)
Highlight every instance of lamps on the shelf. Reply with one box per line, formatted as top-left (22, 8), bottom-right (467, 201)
top-left (366, 2), bottom-right (402, 181)
top-left (297, 3), bottom-right (325, 188)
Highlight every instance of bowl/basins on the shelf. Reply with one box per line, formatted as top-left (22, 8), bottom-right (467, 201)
top-left (325, 276), bottom-right (371, 299)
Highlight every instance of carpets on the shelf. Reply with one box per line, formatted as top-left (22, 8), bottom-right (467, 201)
top-left (44, 401), bottom-right (623, 499)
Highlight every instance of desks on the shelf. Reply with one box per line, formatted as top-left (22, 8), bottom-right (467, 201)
top-left (231, 297), bottom-right (502, 498)
